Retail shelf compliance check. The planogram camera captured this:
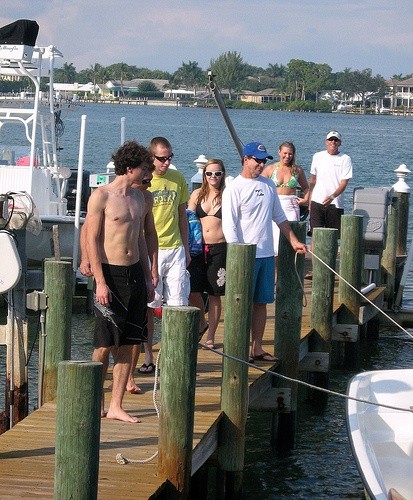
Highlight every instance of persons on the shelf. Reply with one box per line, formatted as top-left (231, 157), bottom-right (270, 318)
top-left (186, 159), bottom-right (227, 351)
top-left (305, 130), bottom-right (353, 281)
top-left (137, 136), bottom-right (192, 374)
top-left (79, 140), bottom-right (160, 424)
top-left (260, 141), bottom-right (310, 286)
top-left (221, 142), bottom-right (308, 362)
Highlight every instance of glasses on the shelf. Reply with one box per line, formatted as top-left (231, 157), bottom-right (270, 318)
top-left (153, 153), bottom-right (174, 162)
top-left (204, 170), bottom-right (224, 177)
top-left (249, 156), bottom-right (268, 164)
top-left (142, 179), bottom-right (151, 184)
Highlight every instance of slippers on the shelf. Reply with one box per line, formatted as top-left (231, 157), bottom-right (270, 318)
top-left (138, 362), bottom-right (156, 374)
top-left (249, 352), bottom-right (280, 362)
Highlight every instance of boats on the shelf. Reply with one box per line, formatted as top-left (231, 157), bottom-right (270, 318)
top-left (345, 363), bottom-right (413, 500)
top-left (0, 18), bottom-right (129, 314)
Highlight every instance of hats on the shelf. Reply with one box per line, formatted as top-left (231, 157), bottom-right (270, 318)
top-left (326, 130), bottom-right (341, 141)
top-left (242, 142), bottom-right (273, 160)
